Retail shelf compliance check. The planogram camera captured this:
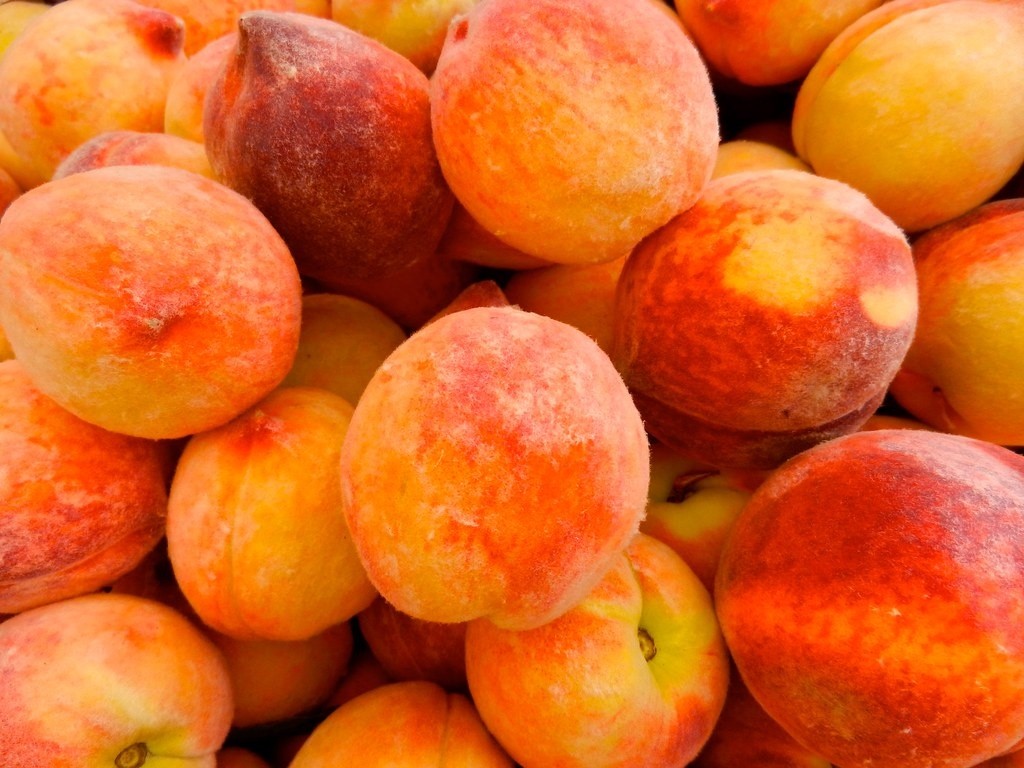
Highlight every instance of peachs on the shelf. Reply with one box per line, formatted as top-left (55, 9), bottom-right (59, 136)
top-left (1, 1), bottom-right (1023, 768)
